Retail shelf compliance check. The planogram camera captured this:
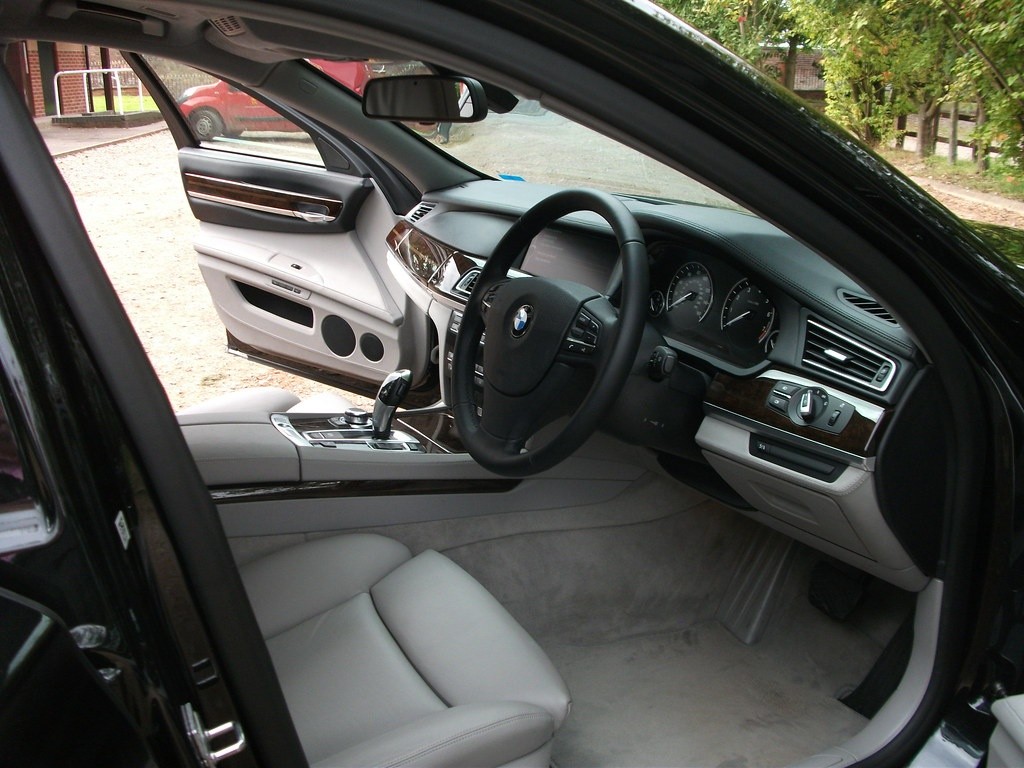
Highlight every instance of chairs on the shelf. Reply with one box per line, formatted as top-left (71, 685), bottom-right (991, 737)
top-left (236, 532), bottom-right (573, 768)
top-left (174, 386), bottom-right (361, 413)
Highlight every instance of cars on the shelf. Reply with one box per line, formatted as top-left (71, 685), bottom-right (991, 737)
top-left (176, 58), bottom-right (440, 143)
top-left (0, 0), bottom-right (1024, 768)
